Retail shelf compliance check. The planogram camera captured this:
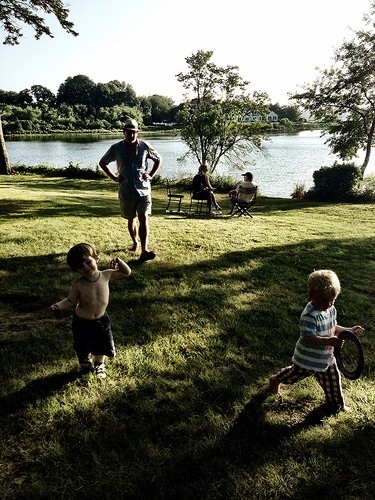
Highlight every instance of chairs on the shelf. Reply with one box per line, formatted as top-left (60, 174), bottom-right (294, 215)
top-left (166, 179), bottom-right (184, 212)
top-left (231, 185), bottom-right (258, 218)
top-left (189, 181), bottom-right (208, 214)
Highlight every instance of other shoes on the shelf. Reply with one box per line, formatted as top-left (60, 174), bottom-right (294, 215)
top-left (81, 361), bottom-right (93, 370)
top-left (216, 206), bottom-right (223, 210)
top-left (140, 251), bottom-right (156, 262)
top-left (342, 406), bottom-right (353, 413)
top-left (95, 363), bottom-right (106, 379)
top-left (270, 376), bottom-right (282, 396)
top-left (131, 242), bottom-right (138, 251)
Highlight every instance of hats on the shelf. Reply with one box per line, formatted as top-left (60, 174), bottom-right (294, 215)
top-left (242, 172), bottom-right (253, 179)
top-left (121, 119), bottom-right (141, 131)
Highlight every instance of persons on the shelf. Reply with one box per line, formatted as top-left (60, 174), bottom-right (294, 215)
top-left (269, 270), bottom-right (365, 412)
top-left (229, 172), bottom-right (255, 215)
top-left (50, 243), bottom-right (131, 379)
top-left (99, 119), bottom-right (161, 261)
top-left (192, 165), bottom-right (222, 210)
